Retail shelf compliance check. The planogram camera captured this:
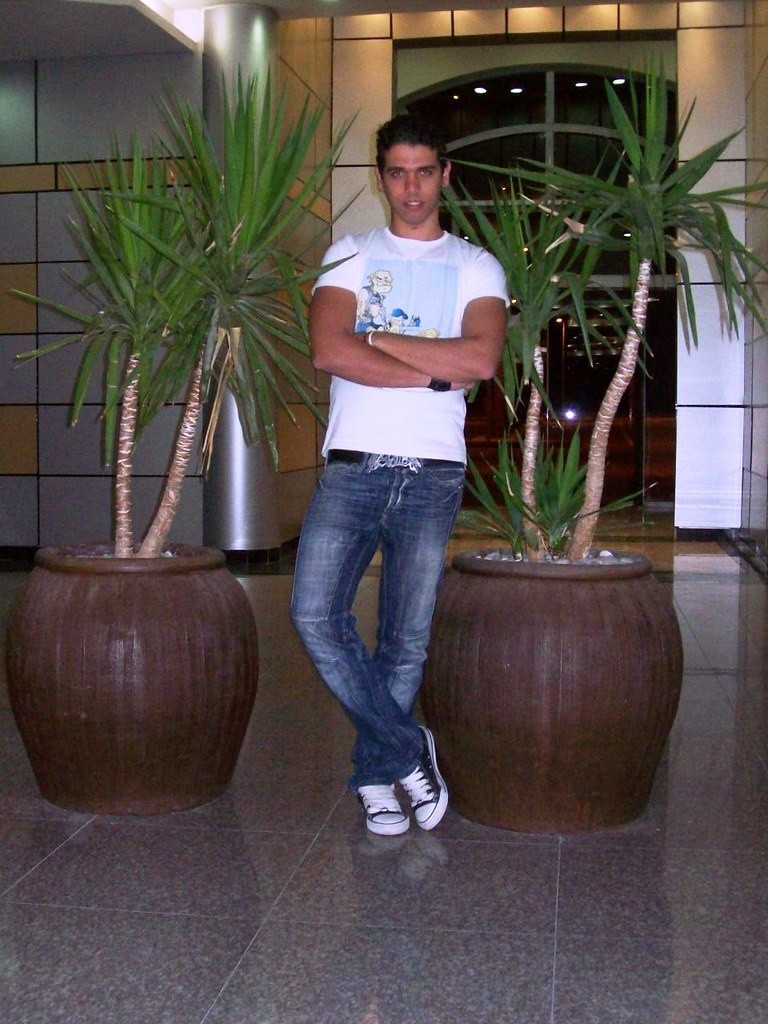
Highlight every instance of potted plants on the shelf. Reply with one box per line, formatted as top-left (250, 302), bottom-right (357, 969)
top-left (0, 47), bottom-right (768, 835)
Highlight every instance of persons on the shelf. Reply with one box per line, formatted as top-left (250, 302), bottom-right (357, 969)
top-left (291, 115), bottom-right (511, 837)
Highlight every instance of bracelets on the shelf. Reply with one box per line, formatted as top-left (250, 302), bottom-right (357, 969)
top-left (363, 332), bottom-right (370, 342)
top-left (428, 377), bottom-right (451, 391)
top-left (368, 331), bottom-right (374, 345)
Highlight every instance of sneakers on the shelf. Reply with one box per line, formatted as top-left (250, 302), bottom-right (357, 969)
top-left (395, 724), bottom-right (449, 829)
top-left (356, 782), bottom-right (409, 835)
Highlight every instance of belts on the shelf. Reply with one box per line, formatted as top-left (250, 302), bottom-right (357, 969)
top-left (326, 448), bottom-right (463, 474)
